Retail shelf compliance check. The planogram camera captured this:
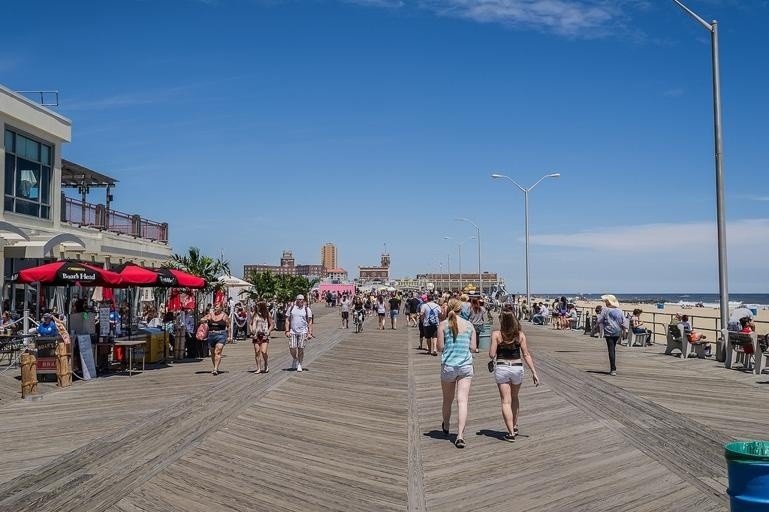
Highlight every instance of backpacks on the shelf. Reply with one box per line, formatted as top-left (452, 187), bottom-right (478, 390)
top-left (426, 304), bottom-right (439, 325)
top-left (461, 305), bottom-right (470, 320)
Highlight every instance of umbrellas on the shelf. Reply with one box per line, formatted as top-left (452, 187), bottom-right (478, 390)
top-left (153, 268), bottom-right (205, 357)
top-left (10, 259), bottom-right (123, 315)
top-left (212, 274), bottom-right (255, 302)
top-left (108, 260), bottom-right (161, 341)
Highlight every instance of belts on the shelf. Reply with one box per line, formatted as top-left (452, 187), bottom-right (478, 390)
top-left (497, 362), bottom-right (522, 365)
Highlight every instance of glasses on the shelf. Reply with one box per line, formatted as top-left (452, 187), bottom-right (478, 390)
top-left (1, 316), bottom-right (6, 319)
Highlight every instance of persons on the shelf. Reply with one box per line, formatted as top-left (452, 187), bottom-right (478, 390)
top-left (249, 301), bottom-right (272, 374)
top-left (521, 295), bottom-right (577, 330)
top-left (437, 298), bottom-right (476, 448)
top-left (728, 309), bottom-right (756, 330)
top-left (38, 312), bottom-right (60, 336)
top-left (343, 288), bottom-right (362, 294)
top-left (110, 303), bottom-right (119, 329)
top-left (0, 312), bottom-right (16, 335)
top-left (439, 289), bottom-right (452, 320)
top-left (739, 318), bottom-right (769, 353)
top-left (489, 311), bottom-right (539, 441)
top-left (312, 290), bottom-right (341, 306)
top-left (350, 298), bottom-right (366, 331)
top-left (460, 294), bottom-right (473, 322)
top-left (364, 289), bottom-right (377, 315)
top-left (469, 299), bottom-right (485, 354)
top-left (671, 312), bottom-right (706, 343)
top-left (376, 296), bottom-right (386, 330)
top-left (388, 293), bottom-right (400, 329)
top-left (286, 295), bottom-right (313, 372)
top-left (590, 294), bottom-right (627, 376)
top-left (200, 303), bottom-right (231, 375)
top-left (403, 288), bottom-right (419, 327)
top-left (595, 305), bottom-right (602, 313)
top-left (340, 295), bottom-right (349, 329)
top-left (631, 309), bottom-right (653, 346)
top-left (419, 296), bottom-right (441, 356)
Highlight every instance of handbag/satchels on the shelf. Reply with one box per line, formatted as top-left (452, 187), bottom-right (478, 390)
top-left (196, 324), bottom-right (209, 341)
top-left (488, 360), bottom-right (495, 372)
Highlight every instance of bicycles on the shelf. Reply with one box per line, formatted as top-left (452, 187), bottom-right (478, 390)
top-left (350, 308), bottom-right (366, 333)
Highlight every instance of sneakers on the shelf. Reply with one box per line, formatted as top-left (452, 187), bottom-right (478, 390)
top-left (514, 427), bottom-right (520, 436)
top-left (212, 368), bottom-right (214, 374)
top-left (441, 421), bottom-right (450, 434)
top-left (455, 438), bottom-right (465, 446)
top-left (297, 363), bottom-right (302, 372)
top-left (610, 370), bottom-right (616, 376)
top-left (213, 368), bottom-right (218, 375)
top-left (434, 349), bottom-right (438, 356)
top-left (503, 433), bottom-right (515, 441)
top-left (292, 359), bottom-right (297, 369)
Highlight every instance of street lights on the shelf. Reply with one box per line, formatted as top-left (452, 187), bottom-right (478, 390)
top-left (491, 173), bottom-right (560, 307)
top-left (455, 217), bottom-right (482, 295)
top-left (442, 237), bottom-right (477, 291)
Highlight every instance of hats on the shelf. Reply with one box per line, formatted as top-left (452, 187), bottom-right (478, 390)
top-left (297, 294), bottom-right (304, 300)
top-left (43, 313), bottom-right (52, 319)
top-left (460, 294), bottom-right (469, 302)
top-left (421, 294), bottom-right (427, 302)
top-left (601, 295), bottom-right (619, 308)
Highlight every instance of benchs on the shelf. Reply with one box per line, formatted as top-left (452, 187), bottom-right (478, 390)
top-left (721, 328), bottom-right (769, 375)
top-left (627, 322), bottom-right (650, 348)
top-left (663, 324), bottom-right (708, 358)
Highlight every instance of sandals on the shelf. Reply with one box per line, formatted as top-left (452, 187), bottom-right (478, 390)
top-left (255, 369), bottom-right (262, 373)
top-left (264, 363), bottom-right (269, 373)
